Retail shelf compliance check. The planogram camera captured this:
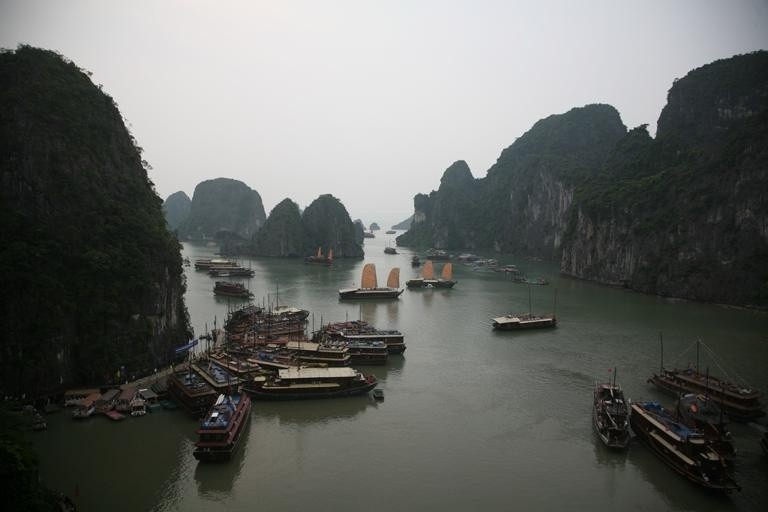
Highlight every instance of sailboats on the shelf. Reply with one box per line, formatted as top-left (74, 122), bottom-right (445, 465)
top-left (406, 261), bottom-right (459, 290)
top-left (339, 262), bottom-right (404, 300)
top-left (304, 246), bottom-right (334, 265)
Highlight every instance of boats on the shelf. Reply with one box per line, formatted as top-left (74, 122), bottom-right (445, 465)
top-left (384, 246), bottom-right (398, 254)
top-left (592, 331), bottom-right (767, 493)
top-left (492, 283), bottom-right (556, 329)
top-left (214, 279), bottom-right (256, 299)
top-left (425, 246), bottom-right (553, 286)
top-left (410, 253), bottom-right (421, 267)
top-left (387, 230), bottom-right (398, 235)
top-left (194, 257), bottom-right (256, 278)
